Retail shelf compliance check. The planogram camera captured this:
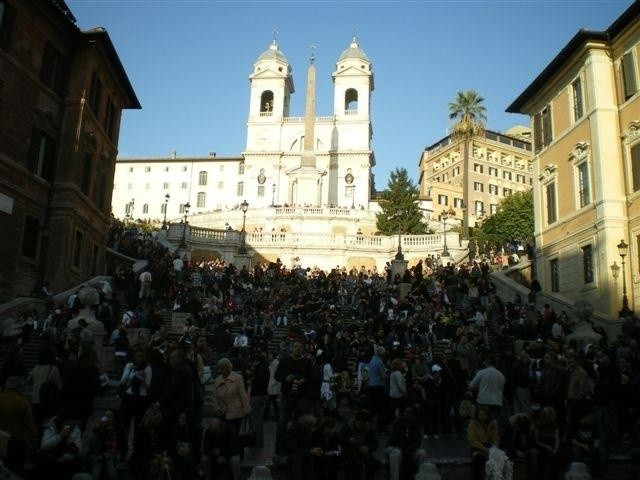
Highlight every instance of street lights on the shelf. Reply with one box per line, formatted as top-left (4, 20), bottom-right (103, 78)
top-left (161, 192), bottom-right (170, 231)
top-left (238, 200), bottom-right (249, 255)
top-left (441, 209), bottom-right (450, 257)
top-left (351, 184), bottom-right (356, 209)
top-left (617, 238), bottom-right (631, 317)
top-left (179, 202), bottom-right (191, 249)
top-left (271, 183), bottom-right (277, 207)
top-left (395, 207), bottom-right (405, 261)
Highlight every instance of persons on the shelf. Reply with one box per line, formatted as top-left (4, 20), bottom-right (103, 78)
top-left (1, 259), bottom-right (639, 479)
top-left (425, 253), bottom-right (443, 268)
top-left (480, 247), bottom-right (519, 265)
top-left (108, 212), bottom-right (187, 263)
